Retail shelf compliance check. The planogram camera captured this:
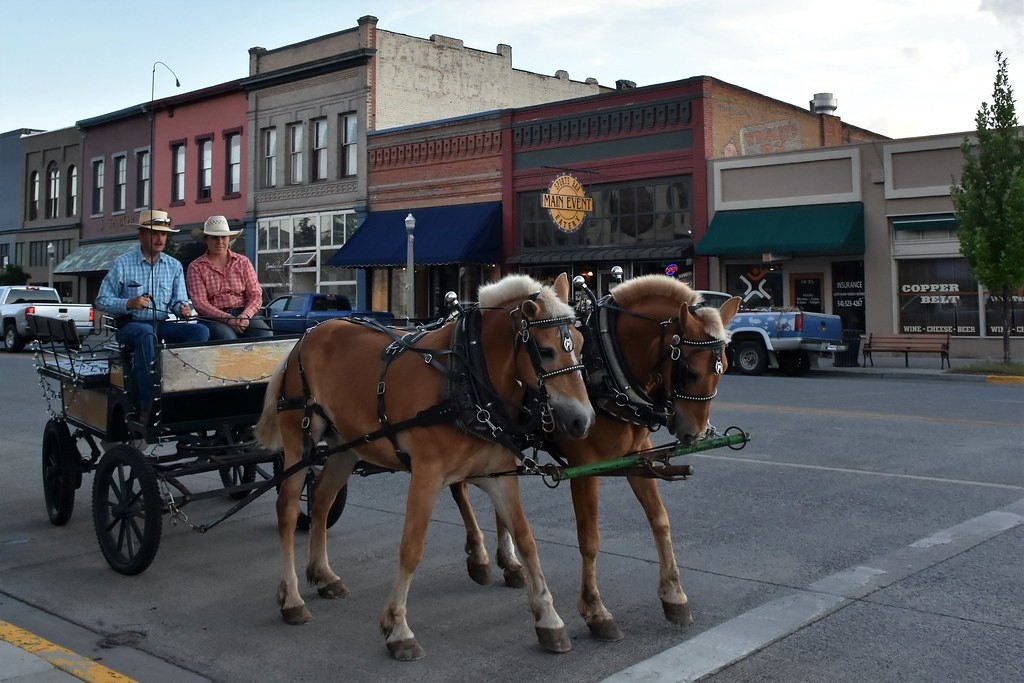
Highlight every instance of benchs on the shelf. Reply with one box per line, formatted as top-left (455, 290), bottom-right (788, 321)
top-left (114, 311), bottom-right (273, 377)
top-left (27, 312), bottom-right (111, 389)
top-left (863, 331), bottom-right (951, 369)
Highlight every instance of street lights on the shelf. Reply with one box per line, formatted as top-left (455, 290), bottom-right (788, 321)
top-left (48, 242), bottom-right (56, 289)
top-left (404, 214), bottom-right (418, 325)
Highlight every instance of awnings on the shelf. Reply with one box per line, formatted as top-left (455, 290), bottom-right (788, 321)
top-left (695, 201), bottom-right (864, 257)
top-left (326, 201), bottom-right (503, 269)
top-left (53, 239), bottom-right (141, 275)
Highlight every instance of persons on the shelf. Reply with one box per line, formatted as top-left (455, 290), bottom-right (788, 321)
top-left (95, 210), bottom-right (209, 425)
top-left (186, 216), bottom-right (273, 340)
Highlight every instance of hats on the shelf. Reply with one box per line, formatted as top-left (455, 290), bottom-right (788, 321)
top-left (191, 216), bottom-right (244, 245)
top-left (126, 210), bottom-right (180, 232)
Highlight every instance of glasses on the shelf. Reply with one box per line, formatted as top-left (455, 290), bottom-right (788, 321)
top-left (142, 218), bottom-right (170, 226)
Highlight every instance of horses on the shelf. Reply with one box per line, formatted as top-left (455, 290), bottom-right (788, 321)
top-left (250, 270), bottom-right (742, 662)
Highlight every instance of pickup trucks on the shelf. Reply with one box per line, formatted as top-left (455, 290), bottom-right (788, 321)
top-left (0, 285), bottom-right (96, 352)
top-left (262, 292), bottom-right (395, 338)
top-left (692, 290), bottom-right (850, 379)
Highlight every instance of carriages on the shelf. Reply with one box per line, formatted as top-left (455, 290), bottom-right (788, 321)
top-left (25, 270), bottom-right (752, 663)
